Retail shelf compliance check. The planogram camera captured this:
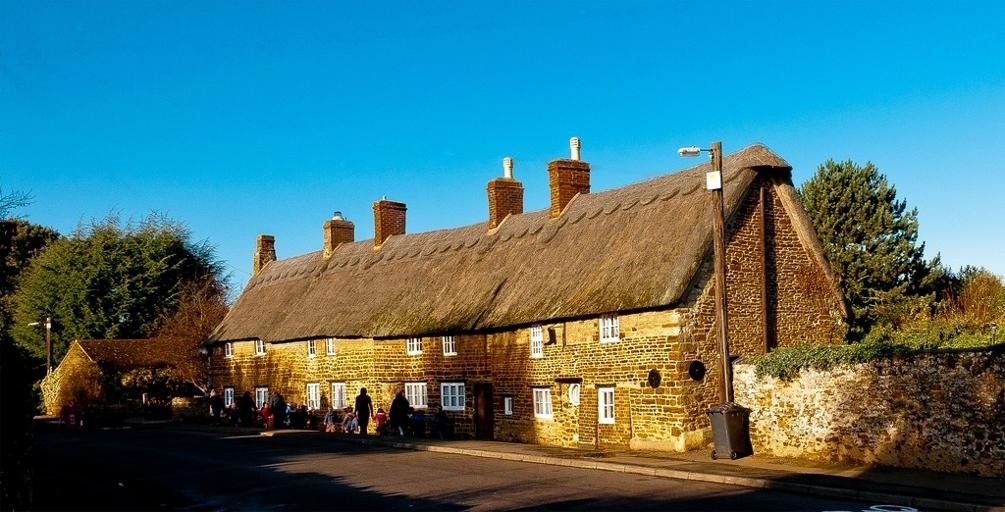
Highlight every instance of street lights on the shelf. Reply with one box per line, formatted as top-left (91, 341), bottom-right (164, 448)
top-left (675, 141), bottom-right (733, 405)
top-left (26, 314), bottom-right (54, 376)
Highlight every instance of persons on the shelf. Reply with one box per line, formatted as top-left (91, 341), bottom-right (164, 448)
top-left (372, 407), bottom-right (388, 441)
top-left (408, 410), bottom-right (427, 439)
top-left (354, 387), bottom-right (375, 438)
top-left (341, 407), bottom-right (358, 435)
top-left (388, 388), bottom-right (413, 437)
top-left (323, 405), bottom-right (338, 433)
top-left (206, 389), bottom-right (298, 431)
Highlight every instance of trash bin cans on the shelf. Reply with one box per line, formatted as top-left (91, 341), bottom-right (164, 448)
top-left (706, 402), bottom-right (748, 460)
